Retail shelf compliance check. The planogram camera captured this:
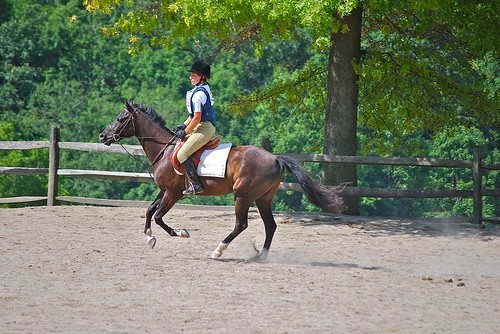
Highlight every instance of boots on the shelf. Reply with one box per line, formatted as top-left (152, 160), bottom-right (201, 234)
top-left (182, 158), bottom-right (203, 194)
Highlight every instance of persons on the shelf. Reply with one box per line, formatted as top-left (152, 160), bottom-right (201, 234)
top-left (175, 60), bottom-right (217, 195)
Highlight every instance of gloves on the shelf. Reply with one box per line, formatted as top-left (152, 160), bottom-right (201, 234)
top-left (175, 130), bottom-right (186, 139)
top-left (173, 123), bottom-right (187, 134)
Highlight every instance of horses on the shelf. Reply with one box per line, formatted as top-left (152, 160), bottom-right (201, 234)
top-left (99, 99), bottom-right (352, 262)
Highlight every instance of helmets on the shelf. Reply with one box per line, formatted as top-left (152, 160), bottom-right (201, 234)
top-left (187, 60), bottom-right (210, 79)
